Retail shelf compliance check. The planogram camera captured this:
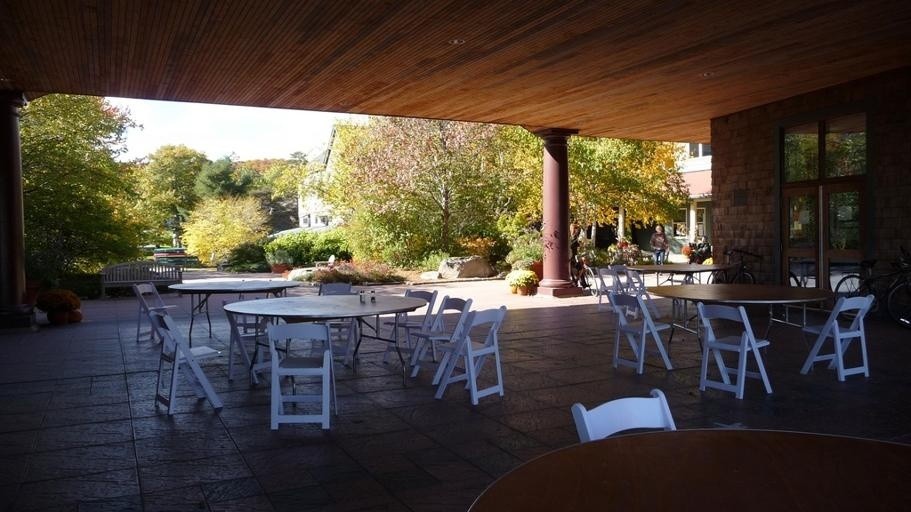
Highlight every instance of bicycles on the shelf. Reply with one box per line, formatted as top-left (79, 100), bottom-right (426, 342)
top-left (569, 249), bottom-right (599, 297)
top-left (834, 245), bottom-right (911, 329)
top-left (708, 248), bottom-right (761, 307)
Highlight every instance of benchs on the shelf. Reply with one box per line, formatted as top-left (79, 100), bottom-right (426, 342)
top-left (98, 258), bottom-right (184, 299)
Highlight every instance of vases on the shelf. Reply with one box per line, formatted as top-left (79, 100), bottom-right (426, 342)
top-left (46, 310), bottom-right (70, 326)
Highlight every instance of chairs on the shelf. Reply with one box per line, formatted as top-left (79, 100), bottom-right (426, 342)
top-left (435, 306), bottom-right (507, 405)
top-left (610, 265), bottom-right (629, 283)
top-left (384, 288), bottom-right (438, 367)
top-left (594, 268), bottom-right (623, 311)
top-left (697, 301), bottom-right (773, 398)
top-left (222, 297), bottom-right (285, 383)
top-left (148, 310), bottom-right (223, 415)
top-left (409, 296), bottom-right (472, 385)
top-left (801, 293), bottom-right (875, 383)
top-left (314, 282), bottom-right (359, 365)
top-left (266, 320), bottom-right (337, 431)
top-left (606, 291), bottom-right (673, 377)
top-left (571, 389), bottom-right (676, 442)
top-left (625, 271), bottom-right (660, 319)
top-left (132, 282), bottom-right (177, 342)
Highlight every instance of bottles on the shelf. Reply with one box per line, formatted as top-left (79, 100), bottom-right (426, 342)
top-left (360, 288), bottom-right (377, 304)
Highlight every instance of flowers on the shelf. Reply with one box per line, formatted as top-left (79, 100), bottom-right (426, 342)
top-left (36, 288), bottom-right (83, 313)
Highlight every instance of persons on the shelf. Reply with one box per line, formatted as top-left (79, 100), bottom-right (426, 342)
top-left (690, 235), bottom-right (711, 263)
top-left (650, 223), bottom-right (668, 276)
top-left (569, 224), bottom-right (591, 288)
